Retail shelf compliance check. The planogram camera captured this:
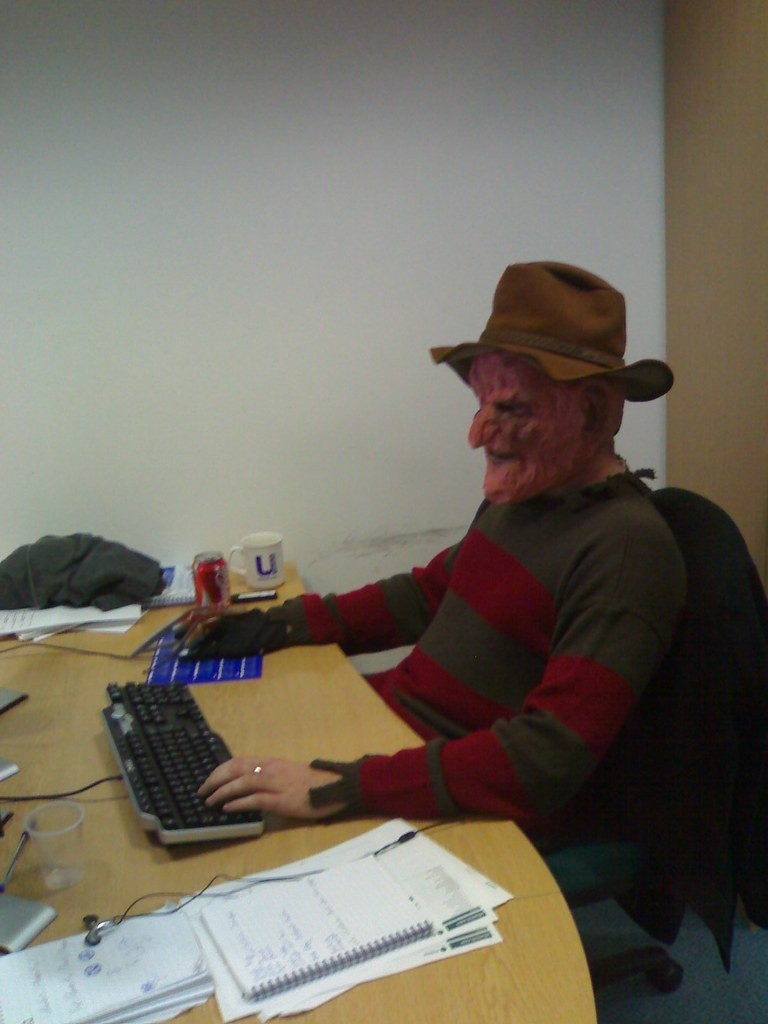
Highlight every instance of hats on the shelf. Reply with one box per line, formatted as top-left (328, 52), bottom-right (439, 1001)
top-left (429, 261), bottom-right (677, 405)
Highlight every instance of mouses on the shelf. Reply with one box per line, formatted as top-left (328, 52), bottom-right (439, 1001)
top-left (178, 628), bottom-right (224, 661)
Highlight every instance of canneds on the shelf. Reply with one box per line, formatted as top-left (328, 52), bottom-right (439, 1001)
top-left (191, 551), bottom-right (230, 611)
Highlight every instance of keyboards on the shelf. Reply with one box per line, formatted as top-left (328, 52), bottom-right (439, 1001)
top-left (101, 681), bottom-right (265, 846)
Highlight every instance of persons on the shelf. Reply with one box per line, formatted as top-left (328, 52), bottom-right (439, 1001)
top-left (175, 262), bottom-right (688, 859)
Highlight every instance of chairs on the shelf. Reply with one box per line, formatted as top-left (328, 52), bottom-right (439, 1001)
top-left (544, 487), bottom-right (768, 995)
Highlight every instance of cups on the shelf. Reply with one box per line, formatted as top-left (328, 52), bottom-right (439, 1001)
top-left (25, 801), bottom-right (86, 890)
top-left (228, 531), bottom-right (286, 590)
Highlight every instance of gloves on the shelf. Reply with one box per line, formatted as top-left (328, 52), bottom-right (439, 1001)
top-left (201, 609), bottom-right (268, 660)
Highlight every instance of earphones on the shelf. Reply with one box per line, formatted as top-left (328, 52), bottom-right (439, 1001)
top-left (84, 920), bottom-right (111, 946)
top-left (82, 914), bottom-right (115, 929)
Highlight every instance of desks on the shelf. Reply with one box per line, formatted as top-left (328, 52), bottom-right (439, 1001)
top-left (0, 559), bottom-right (600, 1024)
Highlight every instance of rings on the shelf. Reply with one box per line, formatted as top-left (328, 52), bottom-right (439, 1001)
top-left (253, 767), bottom-right (261, 776)
